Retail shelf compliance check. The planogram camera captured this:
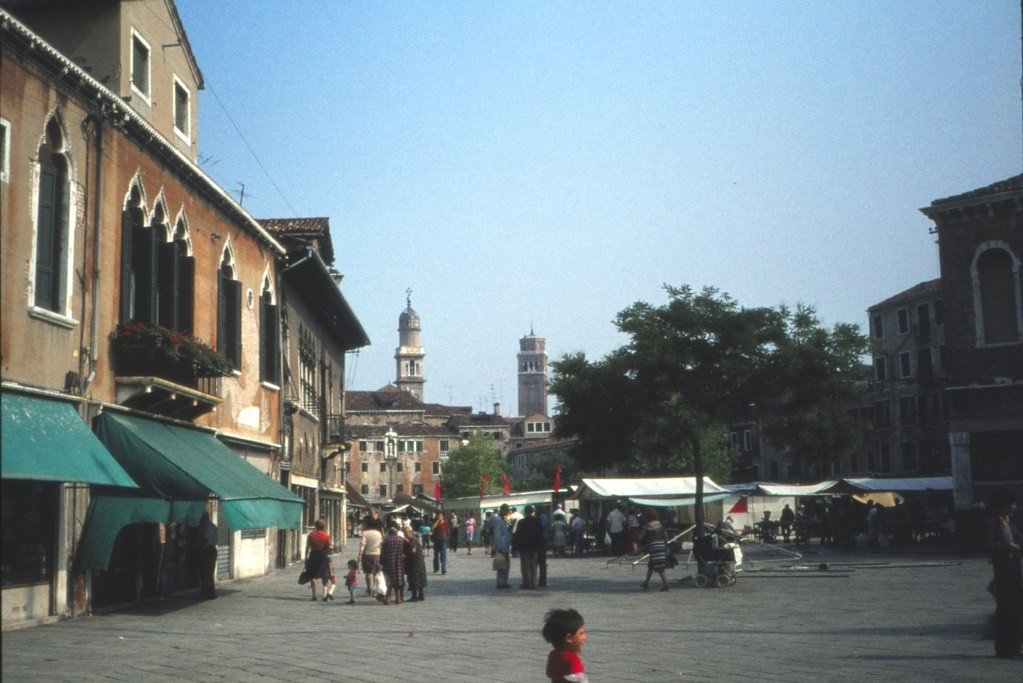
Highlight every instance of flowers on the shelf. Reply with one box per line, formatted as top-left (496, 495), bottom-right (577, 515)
top-left (110, 320), bottom-right (233, 377)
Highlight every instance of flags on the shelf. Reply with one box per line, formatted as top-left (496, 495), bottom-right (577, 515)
top-left (503, 474), bottom-right (509, 493)
top-left (434, 482), bottom-right (440, 500)
top-left (555, 466), bottom-right (561, 491)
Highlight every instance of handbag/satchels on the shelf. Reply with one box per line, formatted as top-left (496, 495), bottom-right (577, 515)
top-left (492, 551), bottom-right (507, 571)
top-left (367, 570), bottom-right (387, 597)
top-left (298, 567), bottom-right (311, 585)
top-left (666, 554), bottom-right (679, 568)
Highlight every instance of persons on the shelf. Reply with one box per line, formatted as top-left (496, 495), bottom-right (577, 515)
top-left (724, 516), bottom-right (738, 534)
top-left (551, 503), bottom-right (639, 558)
top-left (448, 509), bottom-right (498, 555)
top-left (491, 503), bottom-right (548, 590)
top-left (542, 606), bottom-right (587, 683)
top-left (343, 508), bottom-right (431, 604)
top-left (780, 498), bottom-right (900, 547)
top-left (639, 520), bottom-right (670, 592)
top-left (986, 492), bottom-right (1023, 658)
top-left (430, 512), bottom-right (449, 574)
top-left (198, 511), bottom-right (218, 599)
top-left (304, 519), bottom-right (336, 601)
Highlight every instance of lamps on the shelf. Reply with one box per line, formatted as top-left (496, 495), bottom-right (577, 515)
top-left (169, 391), bottom-right (177, 401)
top-left (211, 404), bottom-right (217, 412)
top-left (191, 399), bottom-right (198, 407)
top-left (144, 385), bottom-right (152, 394)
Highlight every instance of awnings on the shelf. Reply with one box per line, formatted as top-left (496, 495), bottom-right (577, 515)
top-left (1, 391), bottom-right (140, 488)
top-left (73, 412), bottom-right (306, 571)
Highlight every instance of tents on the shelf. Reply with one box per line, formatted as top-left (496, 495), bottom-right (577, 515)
top-left (728, 475), bottom-right (954, 541)
top-left (571, 476), bottom-right (730, 555)
top-left (721, 484), bottom-right (795, 531)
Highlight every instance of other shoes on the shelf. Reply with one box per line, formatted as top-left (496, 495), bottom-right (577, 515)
top-left (344, 600), bottom-right (355, 605)
top-left (327, 593), bottom-right (333, 600)
top-left (323, 597), bottom-right (327, 601)
top-left (312, 598), bottom-right (317, 600)
